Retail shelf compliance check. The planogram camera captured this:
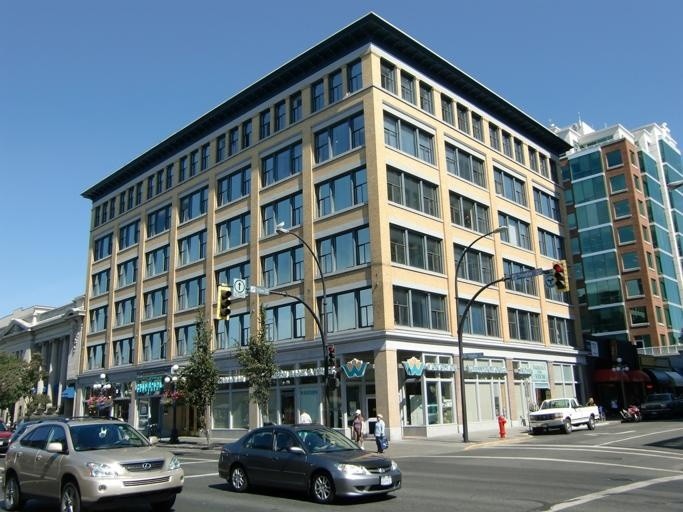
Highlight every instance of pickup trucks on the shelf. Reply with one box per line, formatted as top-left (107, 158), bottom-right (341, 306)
top-left (528, 398), bottom-right (601, 435)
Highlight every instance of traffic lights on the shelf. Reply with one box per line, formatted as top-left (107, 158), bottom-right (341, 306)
top-left (217, 283), bottom-right (231, 322)
top-left (328, 344), bottom-right (336, 366)
top-left (553, 259), bottom-right (570, 292)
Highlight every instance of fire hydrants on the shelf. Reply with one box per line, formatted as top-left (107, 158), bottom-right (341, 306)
top-left (497, 415), bottom-right (507, 439)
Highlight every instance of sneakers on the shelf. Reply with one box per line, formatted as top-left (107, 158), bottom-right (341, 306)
top-left (360, 447), bottom-right (364, 450)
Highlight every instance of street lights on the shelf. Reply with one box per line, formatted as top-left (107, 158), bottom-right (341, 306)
top-left (612, 357), bottom-right (629, 410)
top-left (454, 225), bottom-right (509, 442)
top-left (164, 364), bottom-right (187, 444)
top-left (275, 227), bottom-right (334, 426)
top-left (92, 373), bottom-right (112, 415)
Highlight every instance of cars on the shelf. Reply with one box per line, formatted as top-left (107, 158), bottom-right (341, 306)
top-left (640, 393), bottom-right (681, 421)
top-left (0, 414), bottom-right (184, 512)
top-left (218, 423), bottom-right (403, 505)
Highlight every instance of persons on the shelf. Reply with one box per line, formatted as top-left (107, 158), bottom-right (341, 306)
top-left (300, 409), bottom-right (313, 426)
top-left (374, 413), bottom-right (386, 454)
top-left (350, 407), bottom-right (364, 453)
top-left (585, 397), bottom-right (596, 406)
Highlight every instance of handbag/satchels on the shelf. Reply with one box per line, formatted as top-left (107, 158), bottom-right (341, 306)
top-left (379, 438), bottom-right (388, 449)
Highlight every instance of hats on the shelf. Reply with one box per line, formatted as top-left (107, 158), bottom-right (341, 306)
top-left (356, 409), bottom-right (361, 414)
